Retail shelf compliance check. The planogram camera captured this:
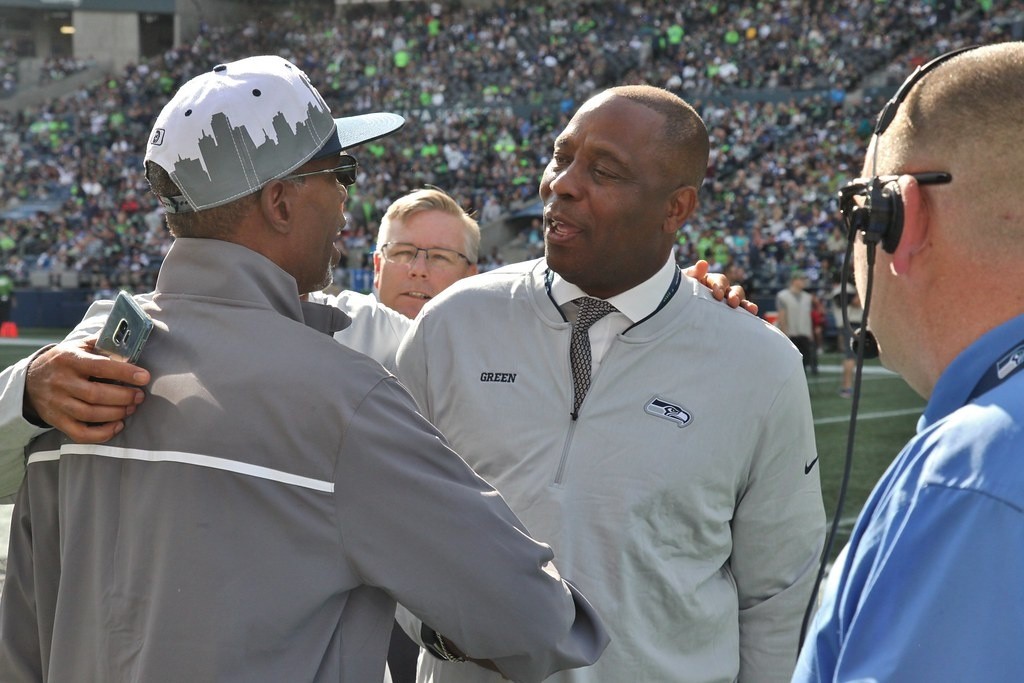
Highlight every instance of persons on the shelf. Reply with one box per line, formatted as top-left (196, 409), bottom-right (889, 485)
top-left (5, 54), bottom-right (610, 683)
top-left (0, 0), bottom-right (1024, 376)
top-left (785, 34), bottom-right (1024, 683)
top-left (3, 188), bottom-right (419, 508)
top-left (368, 187), bottom-right (482, 321)
top-left (390, 84), bottom-right (824, 683)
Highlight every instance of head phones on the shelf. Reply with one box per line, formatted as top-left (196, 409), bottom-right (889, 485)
top-left (839, 43), bottom-right (986, 254)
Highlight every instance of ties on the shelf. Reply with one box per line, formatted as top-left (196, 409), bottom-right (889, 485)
top-left (566, 293), bottom-right (621, 416)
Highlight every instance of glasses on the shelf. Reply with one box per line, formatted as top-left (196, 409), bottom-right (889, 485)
top-left (375, 241), bottom-right (470, 266)
top-left (838, 170), bottom-right (953, 232)
top-left (281, 154), bottom-right (358, 186)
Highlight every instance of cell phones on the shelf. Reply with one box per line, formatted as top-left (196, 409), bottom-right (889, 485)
top-left (84, 290), bottom-right (154, 428)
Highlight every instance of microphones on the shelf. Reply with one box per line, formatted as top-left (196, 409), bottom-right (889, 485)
top-left (842, 205), bottom-right (878, 359)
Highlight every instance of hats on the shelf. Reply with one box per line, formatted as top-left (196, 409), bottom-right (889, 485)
top-left (142, 53), bottom-right (408, 212)
